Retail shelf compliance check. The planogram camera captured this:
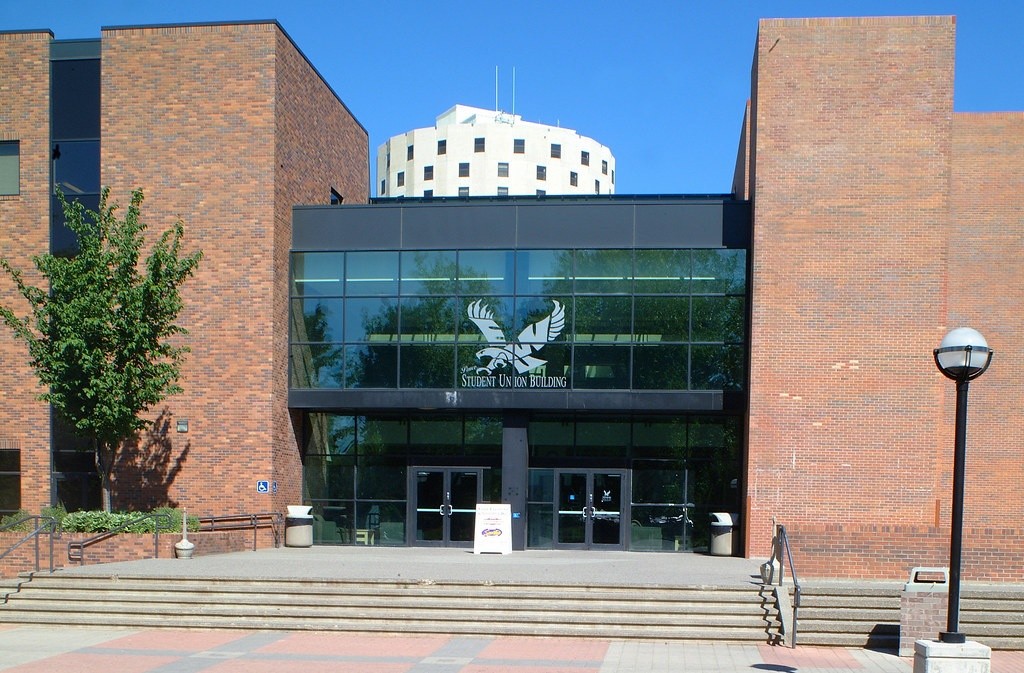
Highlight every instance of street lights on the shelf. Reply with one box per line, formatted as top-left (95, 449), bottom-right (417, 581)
top-left (933, 328), bottom-right (993, 644)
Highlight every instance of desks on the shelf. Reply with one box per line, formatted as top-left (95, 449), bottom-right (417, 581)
top-left (356, 529), bottom-right (376, 545)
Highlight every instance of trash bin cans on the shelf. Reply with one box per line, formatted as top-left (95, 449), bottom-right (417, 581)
top-left (710, 512), bottom-right (740, 556)
top-left (285, 505), bottom-right (314, 547)
top-left (899, 567), bottom-right (960, 656)
